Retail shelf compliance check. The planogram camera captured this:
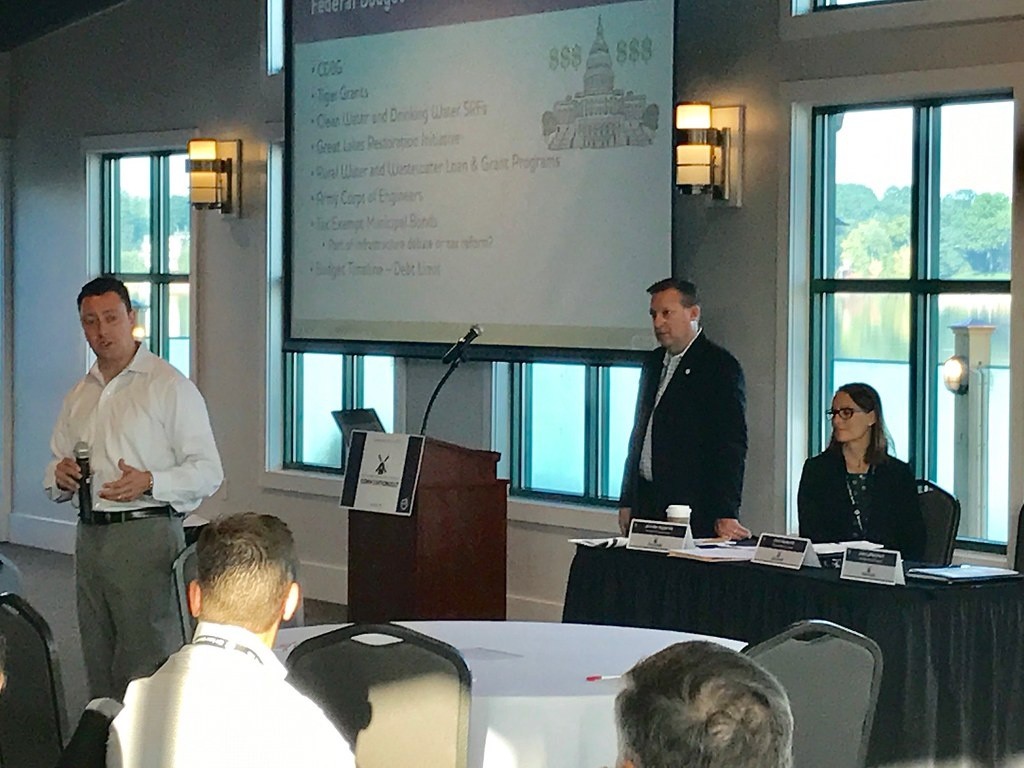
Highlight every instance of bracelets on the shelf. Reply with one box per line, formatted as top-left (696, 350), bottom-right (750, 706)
top-left (148, 472), bottom-right (153, 492)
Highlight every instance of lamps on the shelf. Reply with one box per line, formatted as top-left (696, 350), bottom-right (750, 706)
top-left (187, 137), bottom-right (241, 218)
top-left (672, 99), bottom-right (743, 210)
top-left (944, 355), bottom-right (969, 395)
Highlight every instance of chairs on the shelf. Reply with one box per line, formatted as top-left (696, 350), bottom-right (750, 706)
top-left (912, 478), bottom-right (961, 565)
top-left (738, 619), bottom-right (883, 768)
top-left (0, 590), bottom-right (69, 768)
top-left (285, 620), bottom-right (473, 768)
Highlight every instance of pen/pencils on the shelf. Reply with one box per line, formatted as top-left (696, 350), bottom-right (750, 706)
top-left (283, 642), bottom-right (297, 652)
top-left (586, 674), bottom-right (622, 682)
top-left (835, 541), bottom-right (847, 548)
top-left (949, 565), bottom-right (971, 568)
top-left (725, 542), bottom-right (738, 545)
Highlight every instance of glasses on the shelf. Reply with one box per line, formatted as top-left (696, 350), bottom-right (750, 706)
top-left (825, 407), bottom-right (868, 420)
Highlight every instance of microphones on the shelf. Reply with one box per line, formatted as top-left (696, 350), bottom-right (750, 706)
top-left (72, 441), bottom-right (91, 525)
top-left (442, 324), bottom-right (484, 366)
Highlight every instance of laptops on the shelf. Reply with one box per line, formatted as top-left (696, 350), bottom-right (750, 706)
top-left (333, 409), bottom-right (386, 447)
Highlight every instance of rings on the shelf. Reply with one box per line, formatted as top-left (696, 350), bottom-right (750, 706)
top-left (734, 529), bottom-right (737, 533)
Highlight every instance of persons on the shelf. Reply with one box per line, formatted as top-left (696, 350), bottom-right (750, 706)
top-left (105, 511), bottom-right (356, 768)
top-left (613, 639), bottom-right (793, 768)
top-left (796, 382), bottom-right (929, 562)
top-left (42, 278), bottom-right (224, 701)
top-left (618, 278), bottom-right (753, 542)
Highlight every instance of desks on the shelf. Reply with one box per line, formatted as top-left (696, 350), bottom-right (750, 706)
top-left (562, 545), bottom-right (1024, 768)
top-left (273, 620), bottom-right (751, 768)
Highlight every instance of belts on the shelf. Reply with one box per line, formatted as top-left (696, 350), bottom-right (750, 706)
top-left (80, 504), bottom-right (185, 526)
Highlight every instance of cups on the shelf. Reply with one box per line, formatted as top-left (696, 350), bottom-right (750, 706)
top-left (667, 504), bottom-right (692, 525)
